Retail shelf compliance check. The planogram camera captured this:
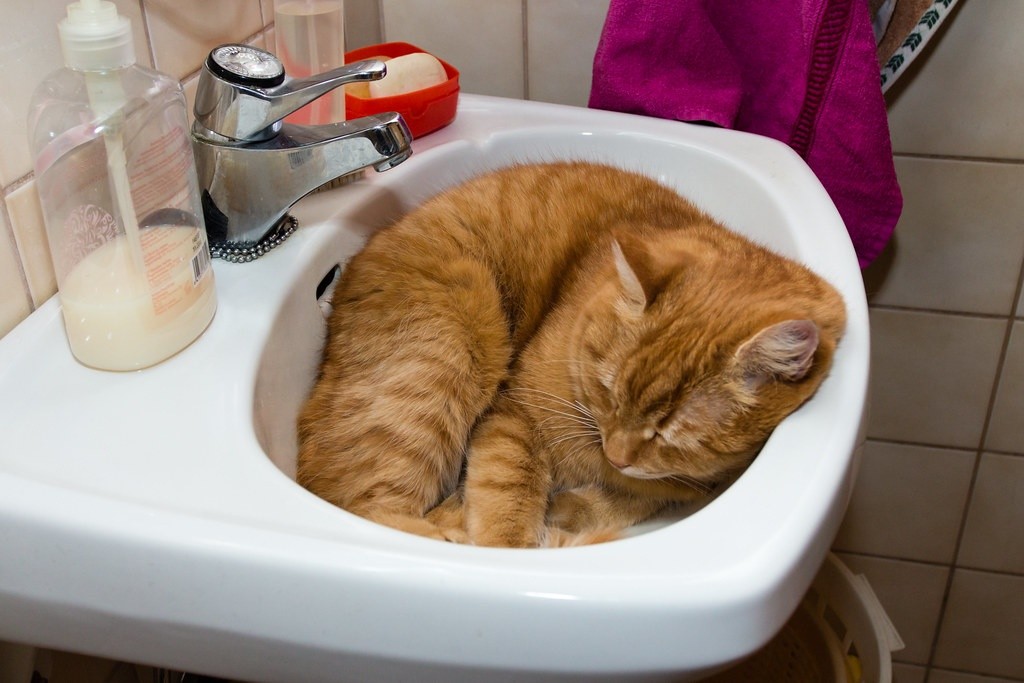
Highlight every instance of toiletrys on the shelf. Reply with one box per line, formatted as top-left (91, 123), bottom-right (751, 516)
top-left (271, 0), bottom-right (349, 128)
top-left (26, 0), bottom-right (219, 375)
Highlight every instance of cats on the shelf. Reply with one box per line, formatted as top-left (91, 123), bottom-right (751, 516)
top-left (292, 159), bottom-right (847, 550)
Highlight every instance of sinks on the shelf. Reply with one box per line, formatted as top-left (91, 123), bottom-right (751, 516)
top-left (1, 59), bottom-right (873, 682)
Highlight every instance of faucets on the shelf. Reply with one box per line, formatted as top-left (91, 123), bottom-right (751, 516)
top-left (168, 43), bottom-right (417, 261)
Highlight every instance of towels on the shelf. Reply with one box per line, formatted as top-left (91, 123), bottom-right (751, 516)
top-left (869, 0), bottom-right (958, 96)
top-left (587, 1), bottom-right (906, 277)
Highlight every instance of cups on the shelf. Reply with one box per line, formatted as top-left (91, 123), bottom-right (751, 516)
top-left (275, 0), bottom-right (346, 142)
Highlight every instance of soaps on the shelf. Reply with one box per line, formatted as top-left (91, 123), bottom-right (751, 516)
top-left (341, 55), bottom-right (393, 101)
top-left (367, 50), bottom-right (447, 103)
